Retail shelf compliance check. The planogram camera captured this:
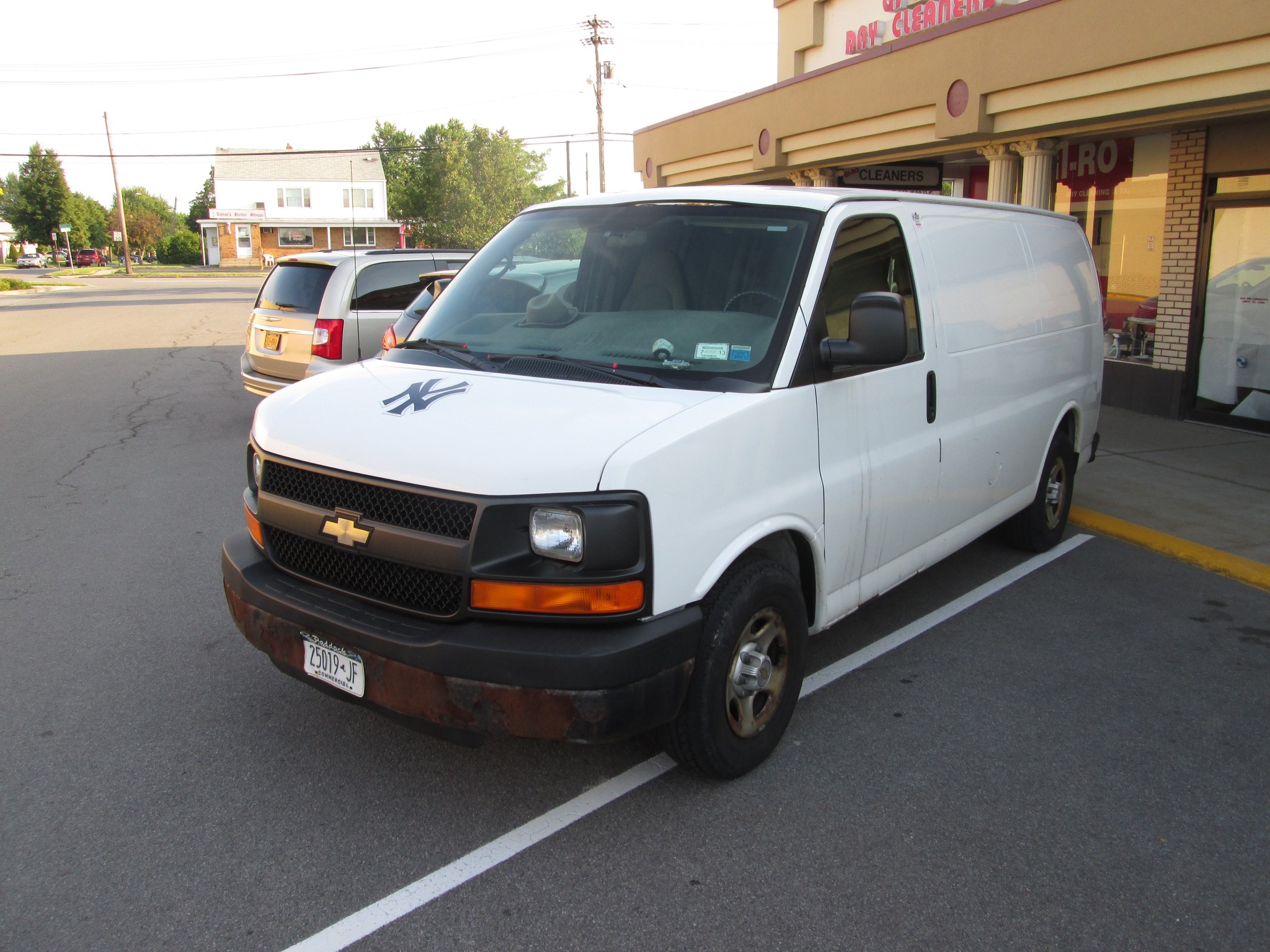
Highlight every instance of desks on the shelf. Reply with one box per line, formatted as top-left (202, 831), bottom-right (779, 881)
top-left (1127, 317), bottom-right (1155, 355)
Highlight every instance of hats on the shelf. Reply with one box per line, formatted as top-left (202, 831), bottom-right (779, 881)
top-left (518, 293), bottom-right (579, 328)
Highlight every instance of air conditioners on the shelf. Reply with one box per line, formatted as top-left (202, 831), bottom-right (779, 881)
top-left (263, 227), bottom-right (272, 234)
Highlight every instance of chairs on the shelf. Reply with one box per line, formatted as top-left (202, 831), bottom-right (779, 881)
top-left (263, 254), bottom-right (274, 266)
top-left (621, 250), bottom-right (688, 311)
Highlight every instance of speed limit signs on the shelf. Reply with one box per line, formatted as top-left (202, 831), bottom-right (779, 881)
top-left (112, 232), bottom-right (122, 241)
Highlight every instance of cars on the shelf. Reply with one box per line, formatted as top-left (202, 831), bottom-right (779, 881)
top-left (104, 255), bottom-right (109, 265)
top-left (64, 251), bottom-right (80, 267)
top-left (16, 253), bottom-right (49, 269)
top-left (1124, 255), bottom-right (1270, 364)
top-left (120, 255), bottom-right (158, 263)
top-left (44, 251), bottom-right (68, 262)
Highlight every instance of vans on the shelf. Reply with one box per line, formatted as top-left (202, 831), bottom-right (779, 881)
top-left (218, 183), bottom-right (1106, 781)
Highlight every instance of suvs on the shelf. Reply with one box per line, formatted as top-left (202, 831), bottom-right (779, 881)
top-left (238, 248), bottom-right (483, 396)
top-left (76, 248), bottom-right (107, 268)
top-left (372, 263), bottom-right (587, 351)
